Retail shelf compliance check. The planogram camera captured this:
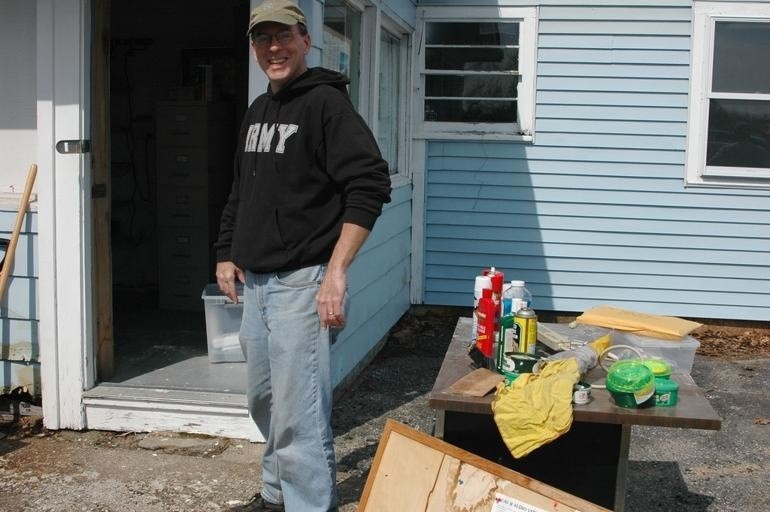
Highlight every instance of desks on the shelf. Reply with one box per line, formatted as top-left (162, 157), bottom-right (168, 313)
top-left (425, 309), bottom-right (723, 512)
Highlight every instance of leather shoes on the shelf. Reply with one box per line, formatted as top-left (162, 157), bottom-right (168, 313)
top-left (230, 492), bottom-right (284, 512)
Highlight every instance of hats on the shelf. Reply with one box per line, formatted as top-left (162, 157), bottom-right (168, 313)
top-left (246, 0), bottom-right (307, 38)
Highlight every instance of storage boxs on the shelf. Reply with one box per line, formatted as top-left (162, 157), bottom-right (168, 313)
top-left (201, 283), bottom-right (248, 364)
top-left (629, 333), bottom-right (701, 376)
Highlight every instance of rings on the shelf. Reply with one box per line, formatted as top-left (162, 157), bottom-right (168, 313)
top-left (223, 280), bottom-right (230, 283)
top-left (327, 312), bottom-right (335, 315)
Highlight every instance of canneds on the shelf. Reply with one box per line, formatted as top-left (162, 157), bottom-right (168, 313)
top-left (503, 351), bottom-right (541, 373)
top-left (571, 382), bottom-right (593, 403)
top-left (501, 370), bottom-right (518, 387)
top-left (606, 359), bottom-right (679, 407)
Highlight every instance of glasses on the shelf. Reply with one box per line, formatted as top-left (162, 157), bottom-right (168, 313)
top-left (251, 29), bottom-right (305, 47)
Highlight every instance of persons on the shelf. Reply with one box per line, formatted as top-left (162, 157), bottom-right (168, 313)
top-left (705, 121), bottom-right (769, 168)
top-left (212, 0), bottom-right (395, 512)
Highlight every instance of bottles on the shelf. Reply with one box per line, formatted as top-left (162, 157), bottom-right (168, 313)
top-left (472, 268), bottom-right (538, 360)
